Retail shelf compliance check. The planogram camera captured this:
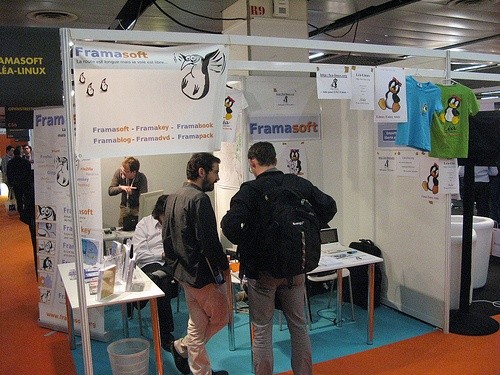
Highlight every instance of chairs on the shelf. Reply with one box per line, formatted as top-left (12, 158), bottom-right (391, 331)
top-left (278, 267), bottom-right (356, 332)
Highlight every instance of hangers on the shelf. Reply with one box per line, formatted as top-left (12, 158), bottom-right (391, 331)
top-left (410, 69), bottom-right (458, 84)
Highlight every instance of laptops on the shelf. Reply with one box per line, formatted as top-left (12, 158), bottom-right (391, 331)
top-left (320, 227), bottom-right (345, 253)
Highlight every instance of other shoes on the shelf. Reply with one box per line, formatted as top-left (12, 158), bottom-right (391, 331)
top-left (145, 272), bottom-right (161, 286)
top-left (158, 332), bottom-right (176, 352)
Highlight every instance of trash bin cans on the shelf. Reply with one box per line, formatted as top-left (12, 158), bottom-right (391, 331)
top-left (106, 338), bottom-right (151, 375)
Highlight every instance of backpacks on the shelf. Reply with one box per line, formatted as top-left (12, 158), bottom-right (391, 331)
top-left (241, 174), bottom-right (322, 279)
top-left (342, 238), bottom-right (381, 309)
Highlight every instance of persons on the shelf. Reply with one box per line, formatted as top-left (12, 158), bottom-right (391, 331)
top-left (107, 157), bottom-right (148, 232)
top-left (126, 194), bottom-right (182, 354)
top-left (0, 144), bottom-right (39, 284)
top-left (220, 141), bottom-right (337, 375)
top-left (161, 152), bottom-right (229, 375)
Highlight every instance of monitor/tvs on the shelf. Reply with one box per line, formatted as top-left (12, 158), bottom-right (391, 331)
top-left (138, 190), bottom-right (164, 223)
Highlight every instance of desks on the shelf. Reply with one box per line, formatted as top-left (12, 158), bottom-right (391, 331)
top-left (56, 258), bottom-right (167, 375)
top-left (223, 241), bottom-right (385, 351)
top-left (103, 227), bottom-right (136, 257)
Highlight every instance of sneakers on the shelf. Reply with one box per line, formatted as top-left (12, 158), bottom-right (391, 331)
top-left (211, 369), bottom-right (228, 375)
top-left (171, 341), bottom-right (191, 375)
